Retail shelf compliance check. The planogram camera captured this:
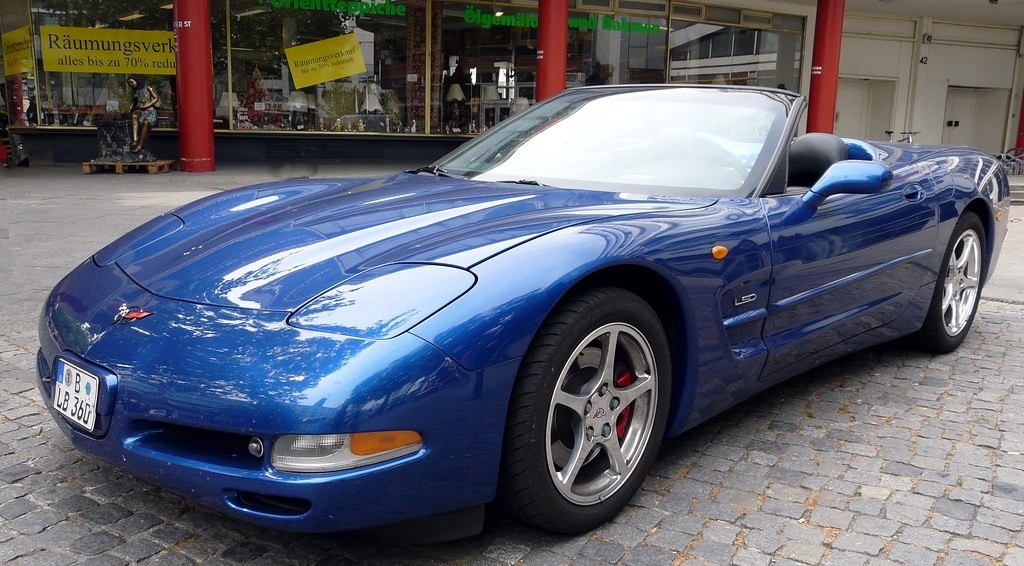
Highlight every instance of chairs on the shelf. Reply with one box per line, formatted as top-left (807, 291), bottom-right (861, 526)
top-left (788, 132), bottom-right (845, 190)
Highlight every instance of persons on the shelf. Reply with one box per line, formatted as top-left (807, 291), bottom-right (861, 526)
top-left (126, 74), bottom-right (159, 152)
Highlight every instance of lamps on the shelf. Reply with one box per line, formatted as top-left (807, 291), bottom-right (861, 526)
top-left (445, 84), bottom-right (466, 134)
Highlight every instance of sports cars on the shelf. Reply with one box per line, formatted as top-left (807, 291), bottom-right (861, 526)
top-left (34, 83), bottom-right (1011, 554)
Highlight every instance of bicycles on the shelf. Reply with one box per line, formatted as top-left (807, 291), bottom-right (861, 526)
top-left (883, 129), bottom-right (921, 144)
